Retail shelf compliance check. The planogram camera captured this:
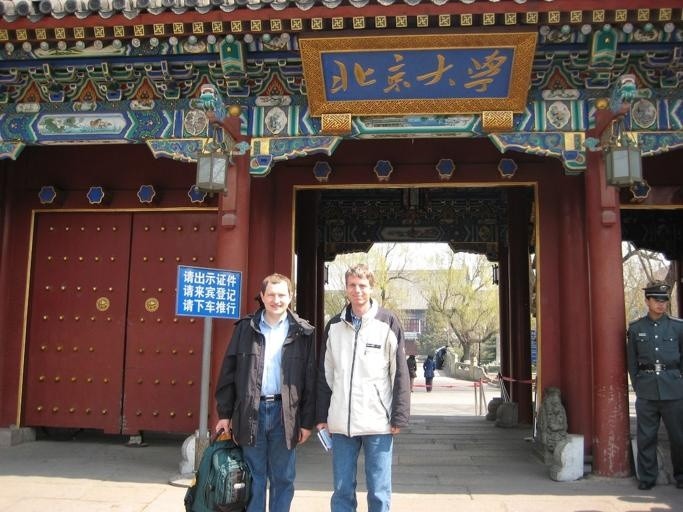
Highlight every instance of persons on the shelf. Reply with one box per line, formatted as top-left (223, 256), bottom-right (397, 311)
top-left (626, 280), bottom-right (683, 491)
top-left (434, 345), bottom-right (448, 367)
top-left (436, 349), bottom-right (447, 370)
top-left (423, 354), bottom-right (436, 392)
top-left (315, 262), bottom-right (412, 512)
top-left (408, 353), bottom-right (418, 392)
top-left (214, 272), bottom-right (318, 512)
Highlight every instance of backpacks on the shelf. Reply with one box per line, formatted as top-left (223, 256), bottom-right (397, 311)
top-left (182, 426), bottom-right (253, 511)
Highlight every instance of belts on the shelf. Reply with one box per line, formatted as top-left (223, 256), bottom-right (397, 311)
top-left (638, 362), bottom-right (680, 372)
top-left (260, 394), bottom-right (281, 400)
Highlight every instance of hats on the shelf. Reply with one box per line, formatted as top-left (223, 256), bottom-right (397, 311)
top-left (641, 282), bottom-right (670, 301)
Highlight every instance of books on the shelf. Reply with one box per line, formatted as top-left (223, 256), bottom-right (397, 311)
top-left (316, 429), bottom-right (333, 452)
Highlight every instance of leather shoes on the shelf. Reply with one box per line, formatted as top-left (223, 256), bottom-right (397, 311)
top-left (639, 481), bottom-right (683, 489)
